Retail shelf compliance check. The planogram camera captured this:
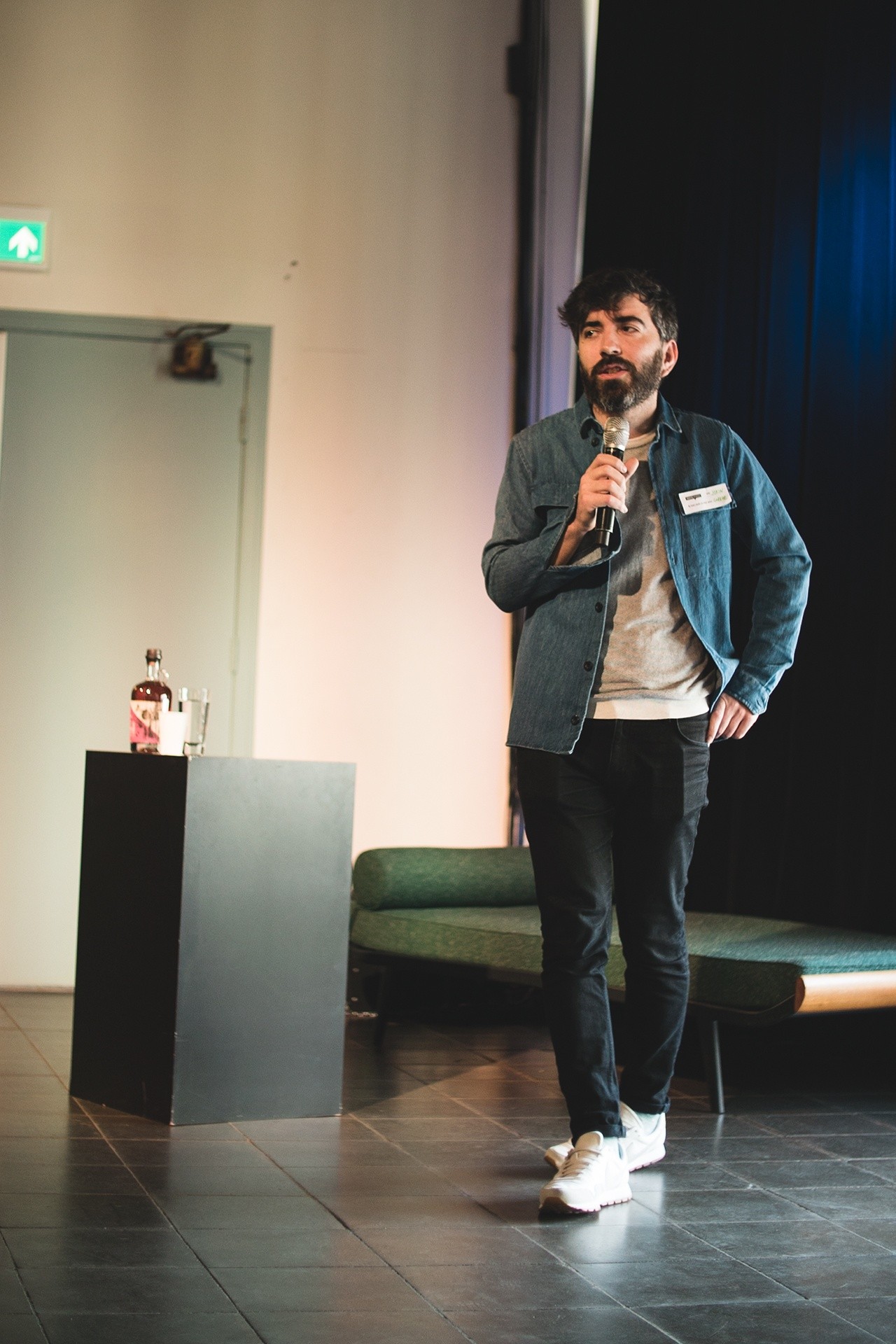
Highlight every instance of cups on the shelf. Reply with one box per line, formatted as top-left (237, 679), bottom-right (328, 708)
top-left (179, 687), bottom-right (210, 757)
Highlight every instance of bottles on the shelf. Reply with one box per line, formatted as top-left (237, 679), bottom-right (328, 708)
top-left (131, 648), bottom-right (173, 755)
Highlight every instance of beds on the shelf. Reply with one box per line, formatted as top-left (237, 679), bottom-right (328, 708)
top-left (348, 841), bottom-right (896, 1115)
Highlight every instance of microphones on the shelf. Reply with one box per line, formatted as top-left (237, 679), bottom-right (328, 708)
top-left (595, 416), bottom-right (630, 547)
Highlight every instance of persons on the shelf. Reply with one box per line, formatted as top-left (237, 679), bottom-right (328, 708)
top-left (480, 268), bottom-right (812, 1213)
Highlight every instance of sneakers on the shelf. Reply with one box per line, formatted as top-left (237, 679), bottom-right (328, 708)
top-left (538, 1131), bottom-right (635, 1218)
top-left (543, 1096), bottom-right (666, 1171)
